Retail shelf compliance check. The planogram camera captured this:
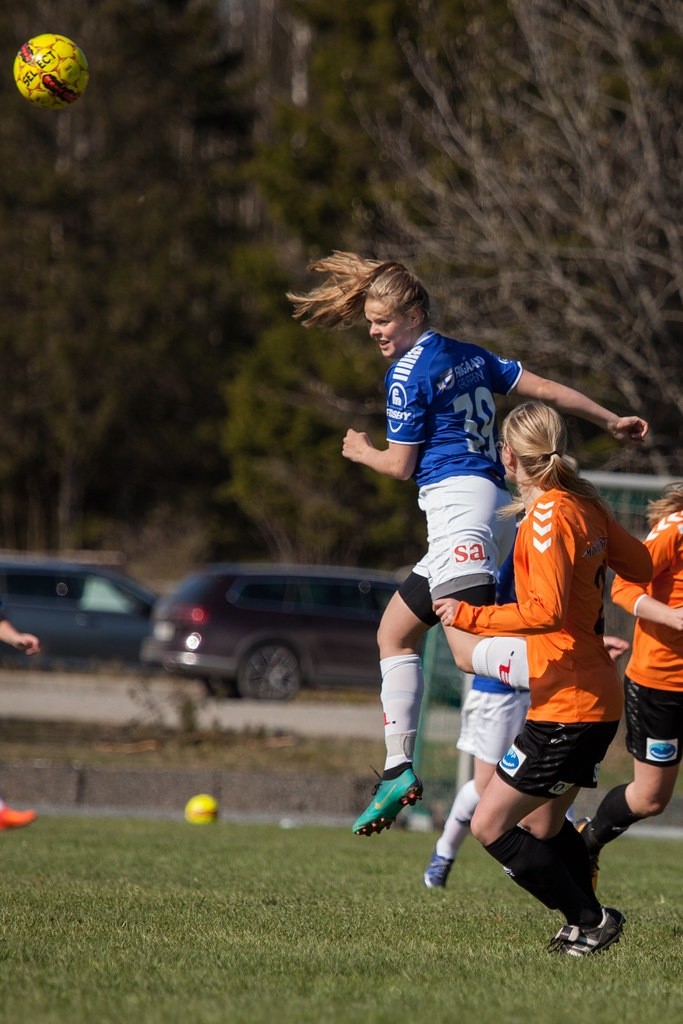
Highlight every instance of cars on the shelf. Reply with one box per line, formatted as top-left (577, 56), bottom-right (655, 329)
top-left (0, 557), bottom-right (176, 672)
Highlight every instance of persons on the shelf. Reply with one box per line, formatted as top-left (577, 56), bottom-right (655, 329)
top-left (574, 481), bottom-right (683, 893)
top-left (432, 402), bottom-right (652, 963)
top-left (0, 591), bottom-right (40, 831)
top-left (284, 249), bottom-right (651, 837)
top-left (424, 505), bottom-right (629, 893)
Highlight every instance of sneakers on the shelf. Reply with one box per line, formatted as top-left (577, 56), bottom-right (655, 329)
top-left (352, 763), bottom-right (423, 837)
top-left (573, 816), bottom-right (602, 892)
top-left (424, 847), bottom-right (453, 889)
top-left (546, 906), bottom-right (624, 960)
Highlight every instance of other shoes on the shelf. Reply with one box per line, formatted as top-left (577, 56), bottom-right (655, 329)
top-left (0, 805), bottom-right (37, 831)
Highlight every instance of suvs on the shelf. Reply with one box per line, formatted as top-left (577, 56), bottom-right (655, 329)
top-left (141, 562), bottom-right (472, 705)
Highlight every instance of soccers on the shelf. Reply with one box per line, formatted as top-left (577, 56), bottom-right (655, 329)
top-left (13, 33), bottom-right (90, 109)
top-left (184, 793), bottom-right (220, 825)
